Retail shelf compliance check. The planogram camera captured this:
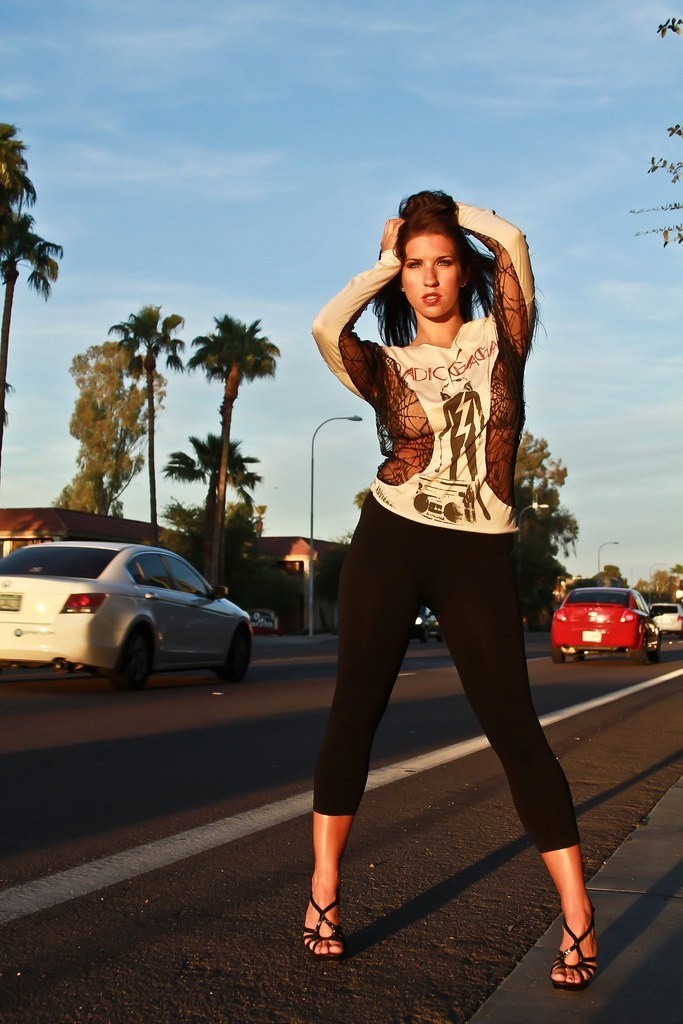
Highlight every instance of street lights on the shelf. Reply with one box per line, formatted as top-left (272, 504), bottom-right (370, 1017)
top-left (308, 415), bottom-right (363, 636)
top-left (519, 504), bottom-right (550, 550)
top-left (649, 563), bottom-right (667, 606)
top-left (598, 541), bottom-right (620, 585)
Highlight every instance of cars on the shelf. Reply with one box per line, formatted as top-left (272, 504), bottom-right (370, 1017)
top-left (550, 588), bottom-right (664, 665)
top-left (649, 603), bottom-right (683, 641)
top-left (0, 540), bottom-right (255, 692)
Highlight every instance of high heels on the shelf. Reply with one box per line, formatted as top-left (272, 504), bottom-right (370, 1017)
top-left (302, 887), bottom-right (346, 960)
top-left (551, 909), bottom-right (598, 990)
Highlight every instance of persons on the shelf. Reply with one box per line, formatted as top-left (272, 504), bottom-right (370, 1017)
top-left (300, 192), bottom-right (600, 990)
